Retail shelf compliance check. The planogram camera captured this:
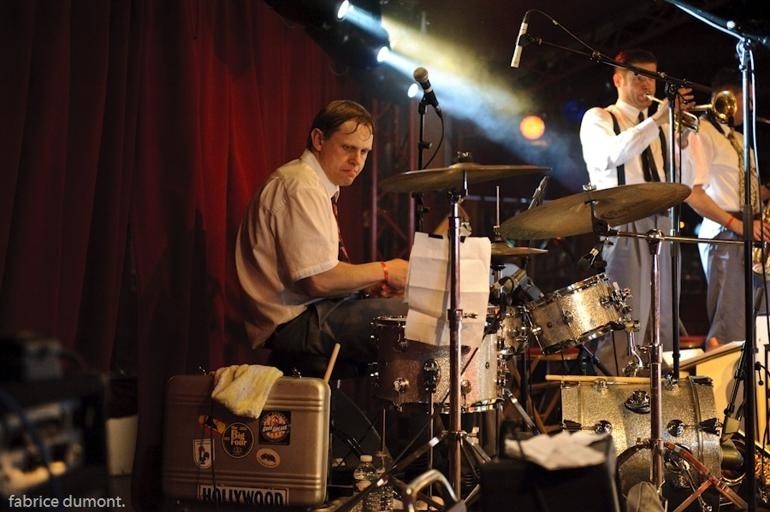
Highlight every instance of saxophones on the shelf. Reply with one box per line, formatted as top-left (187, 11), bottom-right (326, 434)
top-left (727, 118), bottom-right (766, 274)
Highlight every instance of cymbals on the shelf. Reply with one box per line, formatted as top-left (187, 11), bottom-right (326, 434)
top-left (376, 163), bottom-right (551, 193)
top-left (491, 243), bottom-right (549, 257)
top-left (499, 182), bottom-right (693, 241)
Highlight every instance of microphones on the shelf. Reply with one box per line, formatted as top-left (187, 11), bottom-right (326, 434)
top-left (510, 22), bottom-right (528, 68)
top-left (415, 68), bottom-right (442, 117)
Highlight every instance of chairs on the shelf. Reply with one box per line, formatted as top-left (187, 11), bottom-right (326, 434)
top-left (678, 316), bottom-right (708, 348)
top-left (503, 344), bottom-right (581, 435)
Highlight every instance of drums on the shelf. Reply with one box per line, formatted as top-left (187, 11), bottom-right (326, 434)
top-left (521, 273), bottom-right (627, 353)
top-left (370, 306), bottom-right (522, 413)
top-left (561, 378), bottom-right (722, 497)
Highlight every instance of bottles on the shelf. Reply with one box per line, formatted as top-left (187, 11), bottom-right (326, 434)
top-left (352, 454), bottom-right (378, 511)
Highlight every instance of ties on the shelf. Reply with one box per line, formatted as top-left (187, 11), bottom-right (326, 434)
top-left (638, 112), bottom-right (661, 182)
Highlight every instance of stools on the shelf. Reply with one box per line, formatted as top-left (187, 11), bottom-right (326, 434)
top-left (268, 350), bottom-right (370, 485)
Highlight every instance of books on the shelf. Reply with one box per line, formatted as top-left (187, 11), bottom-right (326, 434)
top-left (498, 433), bottom-right (607, 472)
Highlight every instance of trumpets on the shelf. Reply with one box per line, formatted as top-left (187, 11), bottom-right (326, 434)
top-left (644, 89), bottom-right (737, 132)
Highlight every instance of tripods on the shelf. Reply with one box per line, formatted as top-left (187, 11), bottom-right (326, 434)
top-left (334, 202), bottom-right (492, 512)
top-left (503, 430), bottom-right (620, 512)
top-left (615, 243), bottom-right (749, 512)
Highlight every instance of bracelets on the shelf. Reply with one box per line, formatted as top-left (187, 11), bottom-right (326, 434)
top-left (725, 217), bottom-right (736, 229)
top-left (379, 261), bottom-right (390, 285)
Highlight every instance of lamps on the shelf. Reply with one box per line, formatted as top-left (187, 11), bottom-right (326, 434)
top-left (337, 26), bottom-right (389, 66)
top-left (520, 111), bottom-right (552, 148)
top-left (370, 62), bottom-right (419, 101)
top-left (310, 0), bottom-right (352, 23)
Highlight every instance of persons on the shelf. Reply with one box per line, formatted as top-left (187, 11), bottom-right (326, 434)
top-left (576, 44), bottom-right (695, 378)
top-left (680, 62), bottom-right (769, 353)
top-left (228, 94), bottom-right (477, 500)
